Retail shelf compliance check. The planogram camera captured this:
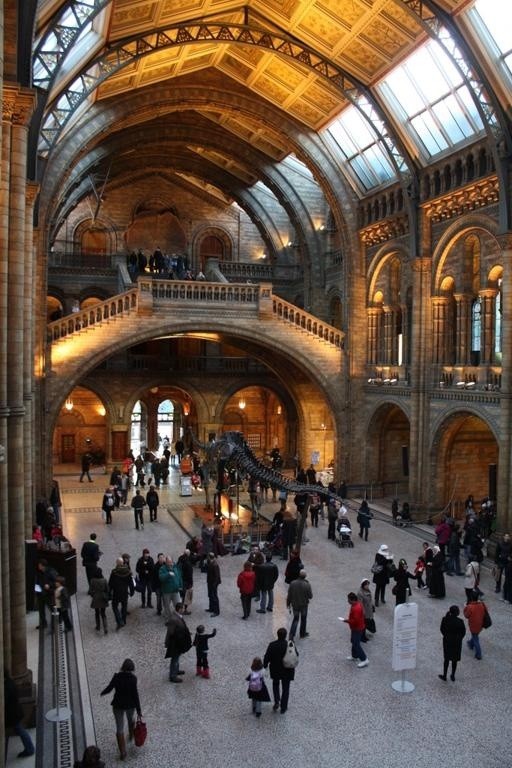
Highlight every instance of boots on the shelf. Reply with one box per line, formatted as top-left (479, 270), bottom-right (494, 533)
top-left (117, 734), bottom-right (126, 760)
top-left (128, 720), bottom-right (135, 739)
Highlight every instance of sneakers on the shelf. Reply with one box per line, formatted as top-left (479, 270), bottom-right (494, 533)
top-left (357, 659), bottom-right (369, 668)
top-left (346, 656), bottom-right (359, 661)
top-left (439, 641), bottom-right (481, 681)
top-left (175, 666), bottom-right (209, 682)
top-left (253, 703), bottom-right (287, 717)
top-left (96, 603), bottom-right (161, 634)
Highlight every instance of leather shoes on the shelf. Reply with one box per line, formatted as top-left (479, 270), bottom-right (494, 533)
top-left (205, 596), bottom-right (273, 619)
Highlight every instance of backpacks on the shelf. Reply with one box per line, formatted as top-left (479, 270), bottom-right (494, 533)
top-left (107, 497), bottom-right (113, 506)
top-left (250, 672), bottom-right (262, 692)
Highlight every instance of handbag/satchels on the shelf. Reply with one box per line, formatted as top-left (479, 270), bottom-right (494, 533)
top-left (371, 563), bottom-right (383, 573)
top-left (483, 612), bottom-right (491, 628)
top-left (283, 640), bottom-right (298, 669)
top-left (135, 717), bottom-right (147, 746)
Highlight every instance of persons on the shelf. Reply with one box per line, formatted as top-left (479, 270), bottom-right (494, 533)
top-left (176, 438), bottom-right (243, 490)
top-left (246, 653), bottom-right (269, 721)
top-left (66, 743), bottom-right (105, 768)
top-left (29, 480), bottom-right (78, 630)
top-left (293, 456), bottom-right (347, 541)
top-left (232, 532), bottom-right (279, 619)
top-left (246, 277), bottom-right (253, 302)
top-left (98, 659), bottom-right (146, 757)
top-left (125, 246), bottom-right (207, 281)
top-left (392, 499), bottom-right (410, 528)
top-left (339, 542), bottom-right (489, 683)
top-left (80, 435), bottom-right (174, 529)
top-left (357, 501), bottom-right (371, 541)
top-left (264, 629), bottom-right (301, 715)
top-left (0, 664), bottom-right (35, 759)
top-left (435, 495), bottom-right (511, 601)
top-left (164, 602), bottom-right (217, 683)
top-left (272, 503), bottom-right (305, 560)
top-left (81, 521), bottom-right (222, 633)
top-left (304, 303), bottom-right (312, 314)
top-left (248, 476), bottom-right (288, 502)
top-left (271, 445), bottom-right (283, 470)
top-left (285, 549), bottom-right (312, 641)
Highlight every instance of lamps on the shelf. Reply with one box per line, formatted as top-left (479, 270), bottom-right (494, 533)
top-left (437, 379), bottom-right (499, 392)
top-left (365, 375), bottom-right (399, 386)
